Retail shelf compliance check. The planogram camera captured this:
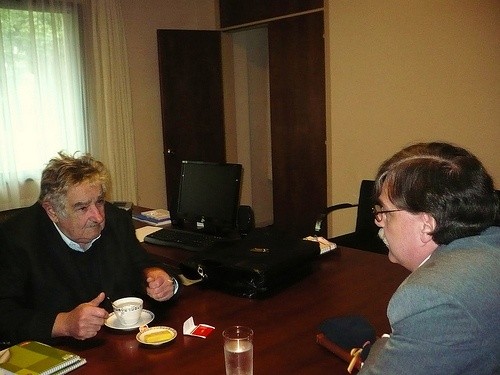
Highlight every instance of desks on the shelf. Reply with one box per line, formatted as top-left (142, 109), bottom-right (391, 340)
top-left (128, 204), bottom-right (245, 271)
top-left (53, 248), bottom-right (406, 375)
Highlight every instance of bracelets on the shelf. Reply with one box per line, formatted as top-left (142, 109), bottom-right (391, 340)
top-left (168, 274), bottom-right (177, 294)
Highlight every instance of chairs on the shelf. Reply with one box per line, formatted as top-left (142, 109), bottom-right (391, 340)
top-left (313, 179), bottom-right (389, 254)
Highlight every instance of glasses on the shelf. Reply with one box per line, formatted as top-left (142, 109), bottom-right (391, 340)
top-left (371, 204), bottom-right (403, 222)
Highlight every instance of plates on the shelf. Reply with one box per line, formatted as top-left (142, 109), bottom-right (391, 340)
top-left (136, 326), bottom-right (178, 345)
top-left (104, 309), bottom-right (155, 331)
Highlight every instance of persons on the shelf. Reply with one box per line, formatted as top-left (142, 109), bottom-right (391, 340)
top-left (357, 142), bottom-right (500, 375)
top-left (0, 152), bottom-right (181, 348)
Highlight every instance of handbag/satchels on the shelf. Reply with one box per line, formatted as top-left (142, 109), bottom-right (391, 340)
top-left (179, 229), bottom-right (320, 297)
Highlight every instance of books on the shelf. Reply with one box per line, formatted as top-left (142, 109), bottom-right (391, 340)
top-left (316, 334), bottom-right (382, 371)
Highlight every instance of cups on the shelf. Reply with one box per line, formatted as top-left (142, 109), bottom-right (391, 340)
top-left (112, 297), bottom-right (143, 326)
top-left (222, 325), bottom-right (254, 375)
